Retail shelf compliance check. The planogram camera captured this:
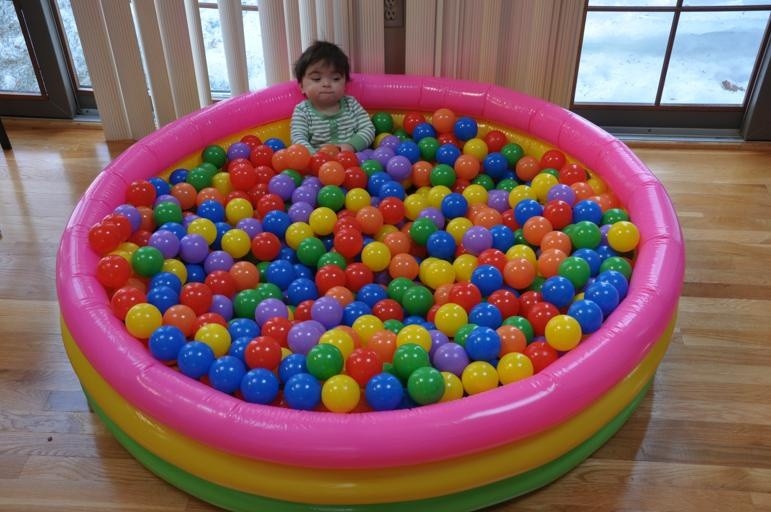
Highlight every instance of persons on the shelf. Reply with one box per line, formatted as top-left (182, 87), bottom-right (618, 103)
top-left (290, 40), bottom-right (376, 154)
